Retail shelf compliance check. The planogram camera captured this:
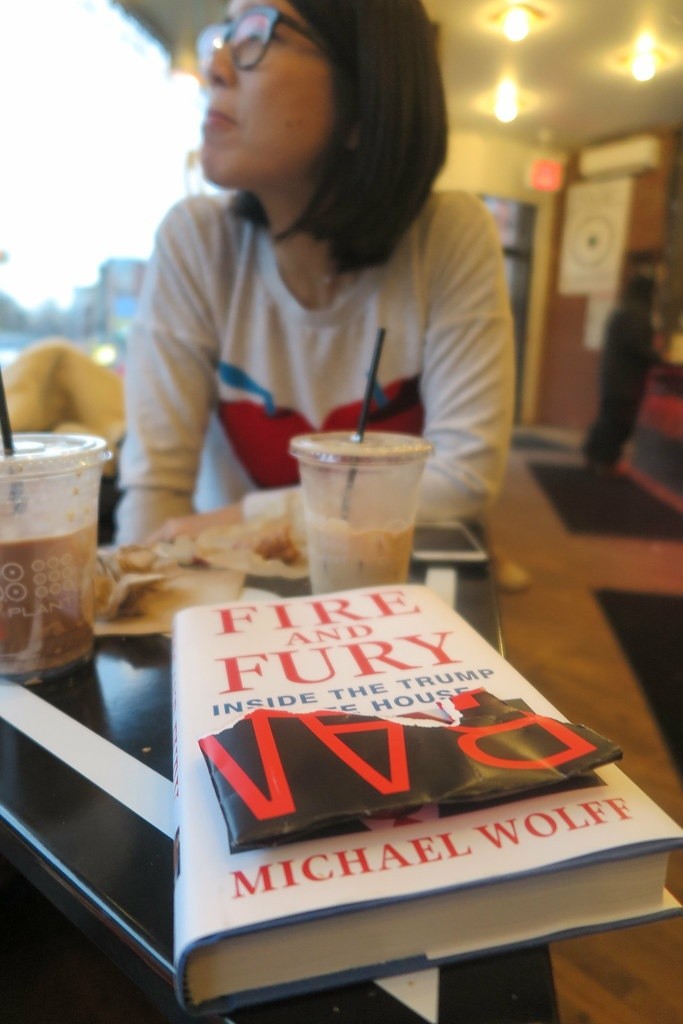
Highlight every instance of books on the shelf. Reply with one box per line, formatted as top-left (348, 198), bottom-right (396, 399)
top-left (172, 583), bottom-right (683, 1018)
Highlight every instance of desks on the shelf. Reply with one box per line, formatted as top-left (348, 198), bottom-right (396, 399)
top-left (0, 514), bottom-right (560, 1024)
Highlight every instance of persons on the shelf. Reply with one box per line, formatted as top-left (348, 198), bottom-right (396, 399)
top-left (114, 0), bottom-right (518, 564)
top-left (583, 248), bottom-right (664, 482)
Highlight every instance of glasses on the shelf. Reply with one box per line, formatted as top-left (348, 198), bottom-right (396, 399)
top-left (196, 6), bottom-right (334, 71)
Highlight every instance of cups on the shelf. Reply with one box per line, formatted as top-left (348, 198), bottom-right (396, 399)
top-left (289, 427), bottom-right (433, 600)
top-left (0, 433), bottom-right (112, 683)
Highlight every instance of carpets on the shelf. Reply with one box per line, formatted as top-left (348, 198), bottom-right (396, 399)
top-left (532, 459), bottom-right (683, 542)
top-left (598, 589), bottom-right (683, 788)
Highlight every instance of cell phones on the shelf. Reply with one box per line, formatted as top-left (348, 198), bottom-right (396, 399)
top-left (411, 522), bottom-right (491, 568)
